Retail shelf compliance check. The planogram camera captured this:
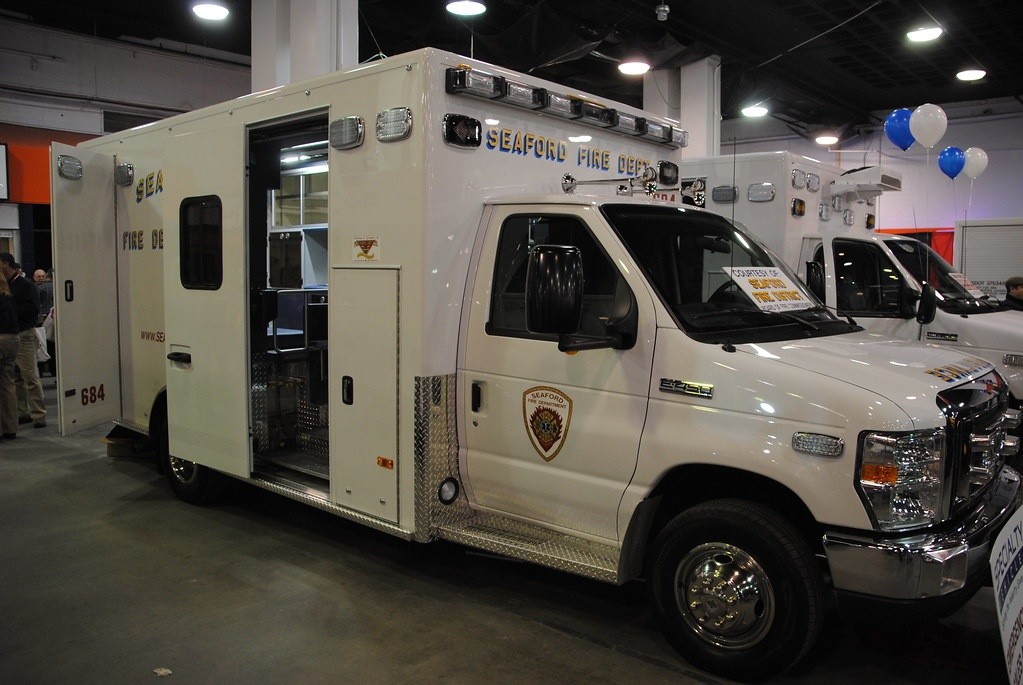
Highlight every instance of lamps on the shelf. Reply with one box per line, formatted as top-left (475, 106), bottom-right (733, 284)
top-left (816, 135), bottom-right (838, 152)
top-left (618, 50), bottom-right (651, 75)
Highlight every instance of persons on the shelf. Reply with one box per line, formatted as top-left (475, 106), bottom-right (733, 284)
top-left (33, 269), bottom-right (56, 378)
top-left (0, 252), bottom-right (47, 440)
top-left (1003, 277), bottom-right (1023, 311)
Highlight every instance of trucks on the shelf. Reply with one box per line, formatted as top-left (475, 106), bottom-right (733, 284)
top-left (681, 151), bottom-right (1023, 403)
top-left (48, 48), bottom-right (1023, 680)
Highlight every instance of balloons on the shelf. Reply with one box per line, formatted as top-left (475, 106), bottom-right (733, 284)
top-left (962, 146), bottom-right (988, 179)
top-left (884, 108), bottom-right (915, 152)
top-left (938, 146), bottom-right (965, 181)
top-left (909, 104), bottom-right (948, 150)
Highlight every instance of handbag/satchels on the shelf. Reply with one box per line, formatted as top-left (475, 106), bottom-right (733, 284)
top-left (32, 327), bottom-right (51, 362)
top-left (41, 307), bottom-right (55, 343)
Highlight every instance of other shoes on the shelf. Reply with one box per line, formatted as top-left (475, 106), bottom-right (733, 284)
top-left (2, 433), bottom-right (16, 438)
top-left (19, 414), bottom-right (33, 424)
top-left (32, 417), bottom-right (46, 426)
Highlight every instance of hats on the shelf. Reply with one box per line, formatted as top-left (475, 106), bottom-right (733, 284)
top-left (1006, 277), bottom-right (1023, 288)
